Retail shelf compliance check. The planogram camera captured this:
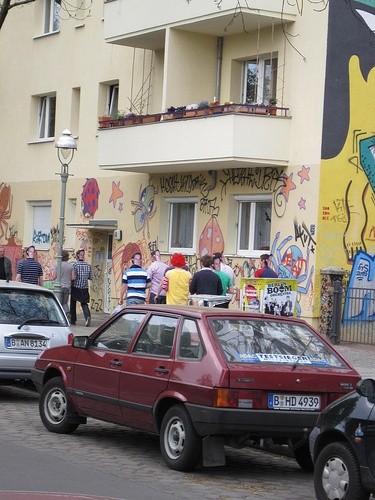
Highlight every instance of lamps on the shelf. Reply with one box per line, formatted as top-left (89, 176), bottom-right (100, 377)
top-left (114, 229), bottom-right (123, 241)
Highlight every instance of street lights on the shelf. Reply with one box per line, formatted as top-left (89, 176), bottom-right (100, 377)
top-left (53, 130), bottom-right (79, 315)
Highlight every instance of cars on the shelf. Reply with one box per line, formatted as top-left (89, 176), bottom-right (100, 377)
top-left (0, 280), bottom-right (74, 383)
top-left (307, 378), bottom-right (375, 500)
top-left (31, 303), bottom-right (363, 472)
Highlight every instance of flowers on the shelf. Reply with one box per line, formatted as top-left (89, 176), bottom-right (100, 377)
top-left (126, 112), bottom-right (135, 119)
top-left (167, 98), bottom-right (234, 112)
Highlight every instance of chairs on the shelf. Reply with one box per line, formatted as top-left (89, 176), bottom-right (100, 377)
top-left (151, 326), bottom-right (193, 358)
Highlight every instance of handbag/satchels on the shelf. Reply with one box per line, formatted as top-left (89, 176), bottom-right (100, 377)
top-left (111, 305), bottom-right (123, 317)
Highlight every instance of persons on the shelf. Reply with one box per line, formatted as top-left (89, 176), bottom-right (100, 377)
top-left (154, 252), bottom-right (192, 305)
top-left (52, 250), bottom-right (76, 321)
top-left (213, 257), bottom-right (221, 272)
top-left (0, 246), bottom-right (12, 280)
top-left (264, 296), bottom-right (292, 317)
top-left (147, 250), bottom-right (169, 337)
top-left (27, 246), bottom-right (35, 258)
top-left (212, 257), bottom-right (234, 308)
top-left (249, 254), bottom-right (278, 278)
top-left (189, 256), bottom-right (223, 306)
top-left (17, 247), bottom-right (43, 287)
top-left (78, 250), bottom-right (85, 260)
top-left (118, 253), bottom-right (150, 335)
top-left (132, 253), bottom-right (141, 265)
top-left (69, 249), bottom-right (93, 327)
top-left (213, 253), bottom-right (234, 281)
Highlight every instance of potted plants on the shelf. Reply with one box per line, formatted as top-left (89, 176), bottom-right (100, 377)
top-left (268, 98), bottom-right (277, 115)
top-left (111, 112), bottom-right (124, 128)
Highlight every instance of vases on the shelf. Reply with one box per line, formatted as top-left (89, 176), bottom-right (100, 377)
top-left (97, 120), bottom-right (111, 127)
top-left (124, 114), bottom-right (160, 125)
top-left (163, 104), bottom-right (267, 120)
